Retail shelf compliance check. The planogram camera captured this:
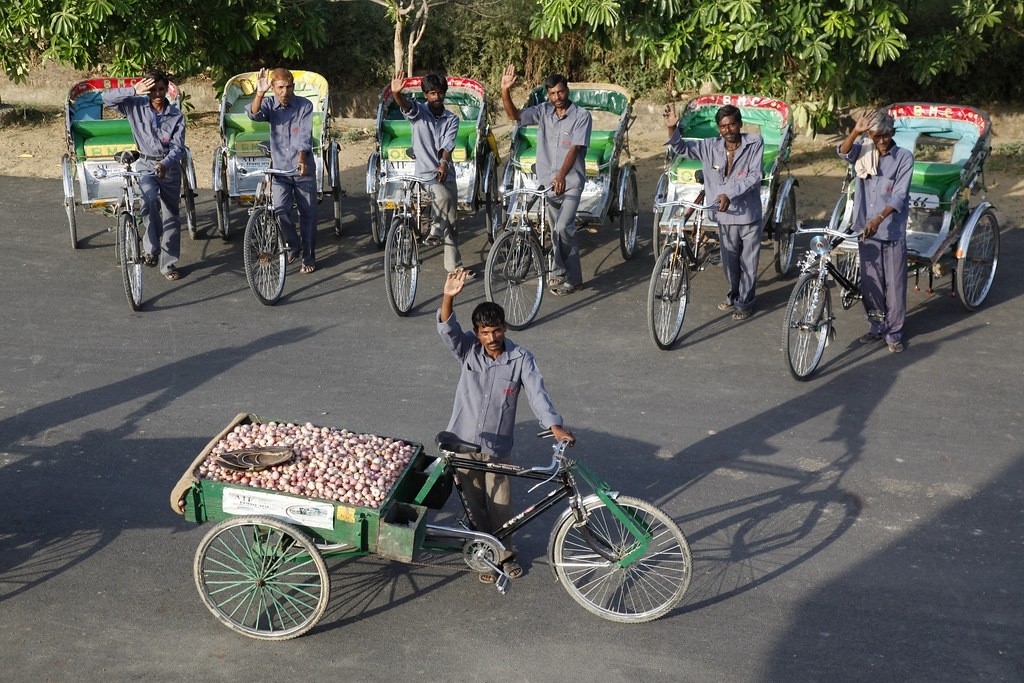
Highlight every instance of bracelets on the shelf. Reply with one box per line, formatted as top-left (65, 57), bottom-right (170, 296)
top-left (256, 93), bottom-right (264, 99)
top-left (440, 157), bottom-right (451, 166)
top-left (879, 214), bottom-right (884, 219)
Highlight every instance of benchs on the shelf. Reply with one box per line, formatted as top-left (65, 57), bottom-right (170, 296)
top-left (75, 119), bottom-right (138, 181)
top-left (382, 120), bottom-right (477, 178)
top-left (672, 139), bottom-right (780, 199)
top-left (222, 112), bottom-right (322, 183)
top-left (516, 126), bottom-right (611, 191)
top-left (841, 159), bottom-right (963, 229)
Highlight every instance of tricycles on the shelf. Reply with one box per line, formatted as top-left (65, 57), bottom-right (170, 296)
top-left (367, 76), bottom-right (506, 317)
top-left (61, 75), bottom-right (198, 309)
top-left (474, 81), bottom-right (645, 324)
top-left (789, 103), bottom-right (1003, 380)
top-left (211, 69), bottom-right (346, 305)
top-left (166, 411), bottom-right (689, 641)
top-left (641, 92), bottom-right (803, 350)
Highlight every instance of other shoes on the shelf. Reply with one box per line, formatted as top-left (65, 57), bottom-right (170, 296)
top-left (547, 274), bottom-right (570, 288)
top-left (718, 300), bottom-right (735, 310)
top-left (462, 269), bottom-right (477, 279)
top-left (551, 282), bottom-right (576, 296)
top-left (426, 234), bottom-right (440, 243)
top-left (733, 307), bottom-right (753, 320)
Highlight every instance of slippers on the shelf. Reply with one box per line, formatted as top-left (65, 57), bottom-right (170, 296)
top-left (886, 341), bottom-right (903, 353)
top-left (285, 248), bottom-right (300, 264)
top-left (478, 570), bottom-right (497, 583)
top-left (503, 557), bottom-right (523, 577)
top-left (145, 252), bottom-right (158, 268)
top-left (860, 332), bottom-right (880, 343)
top-left (300, 260), bottom-right (315, 273)
top-left (164, 264), bottom-right (179, 280)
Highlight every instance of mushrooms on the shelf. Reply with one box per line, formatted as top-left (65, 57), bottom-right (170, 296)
top-left (175, 422), bottom-right (416, 514)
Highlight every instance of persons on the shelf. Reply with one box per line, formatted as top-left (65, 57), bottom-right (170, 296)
top-left (501, 64), bottom-right (592, 296)
top-left (247, 67), bottom-right (317, 274)
top-left (837, 109), bottom-right (914, 351)
top-left (391, 67), bottom-right (477, 279)
top-left (437, 267), bottom-right (577, 584)
top-left (663, 104), bottom-right (764, 320)
top-left (102, 72), bottom-right (187, 279)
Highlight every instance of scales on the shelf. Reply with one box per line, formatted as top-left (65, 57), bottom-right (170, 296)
top-left (214, 446), bottom-right (295, 471)
